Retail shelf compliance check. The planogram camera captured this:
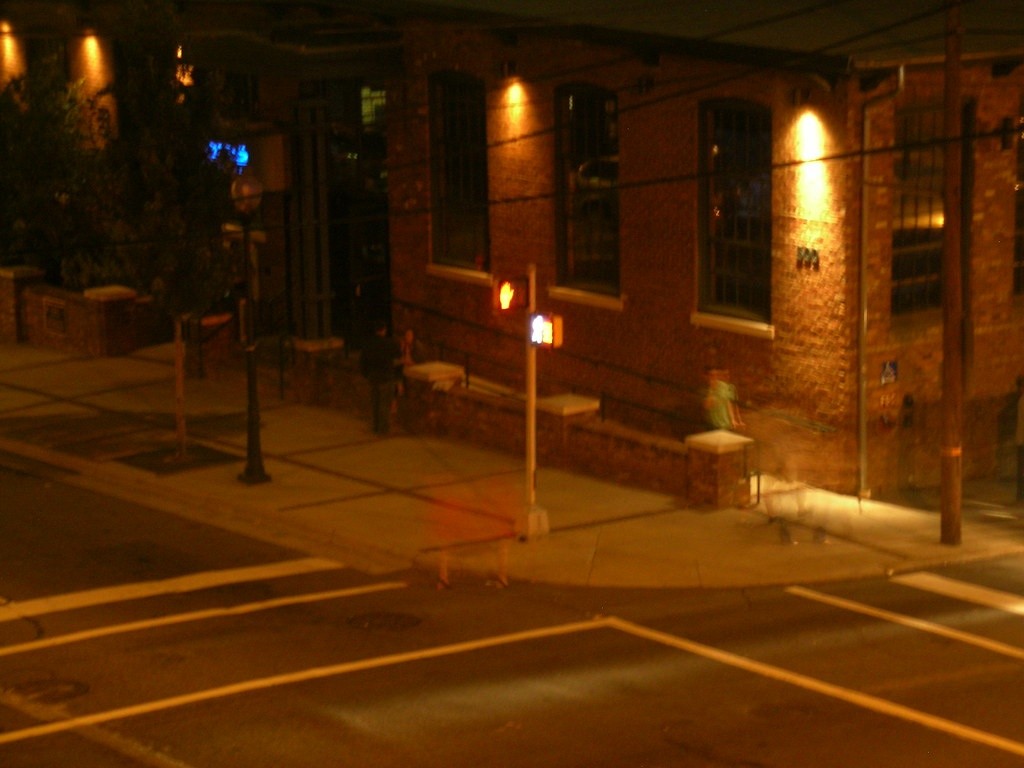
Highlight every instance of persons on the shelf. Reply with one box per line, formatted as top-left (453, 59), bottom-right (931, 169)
top-left (361, 321), bottom-right (405, 437)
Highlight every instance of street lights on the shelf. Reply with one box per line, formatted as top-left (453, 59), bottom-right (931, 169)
top-left (229, 164), bottom-right (273, 486)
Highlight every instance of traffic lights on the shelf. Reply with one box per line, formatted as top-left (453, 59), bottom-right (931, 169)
top-left (491, 275), bottom-right (528, 314)
top-left (529, 309), bottom-right (564, 350)
